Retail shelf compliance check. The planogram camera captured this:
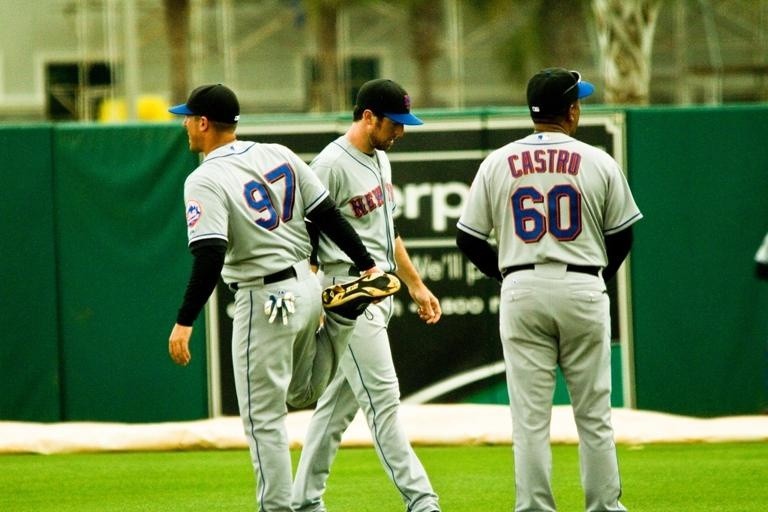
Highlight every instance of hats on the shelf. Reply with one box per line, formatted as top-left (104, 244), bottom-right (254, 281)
top-left (525, 67), bottom-right (595, 114)
top-left (356, 79), bottom-right (424, 125)
top-left (168, 84), bottom-right (240, 124)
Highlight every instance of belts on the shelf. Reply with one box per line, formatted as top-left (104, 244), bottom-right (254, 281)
top-left (347, 264), bottom-right (359, 278)
top-left (230, 269), bottom-right (295, 289)
top-left (503, 264), bottom-right (599, 276)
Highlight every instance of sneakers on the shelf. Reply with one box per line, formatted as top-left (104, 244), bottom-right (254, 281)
top-left (320, 271), bottom-right (401, 321)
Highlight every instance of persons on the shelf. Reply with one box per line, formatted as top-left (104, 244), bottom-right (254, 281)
top-left (291, 78), bottom-right (443, 512)
top-left (454, 68), bottom-right (643, 512)
top-left (168, 84), bottom-right (403, 512)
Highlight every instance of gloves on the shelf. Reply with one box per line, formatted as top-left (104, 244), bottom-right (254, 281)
top-left (264, 291), bottom-right (296, 326)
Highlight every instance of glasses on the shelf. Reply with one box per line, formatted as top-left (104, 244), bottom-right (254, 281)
top-left (561, 70), bottom-right (582, 96)
